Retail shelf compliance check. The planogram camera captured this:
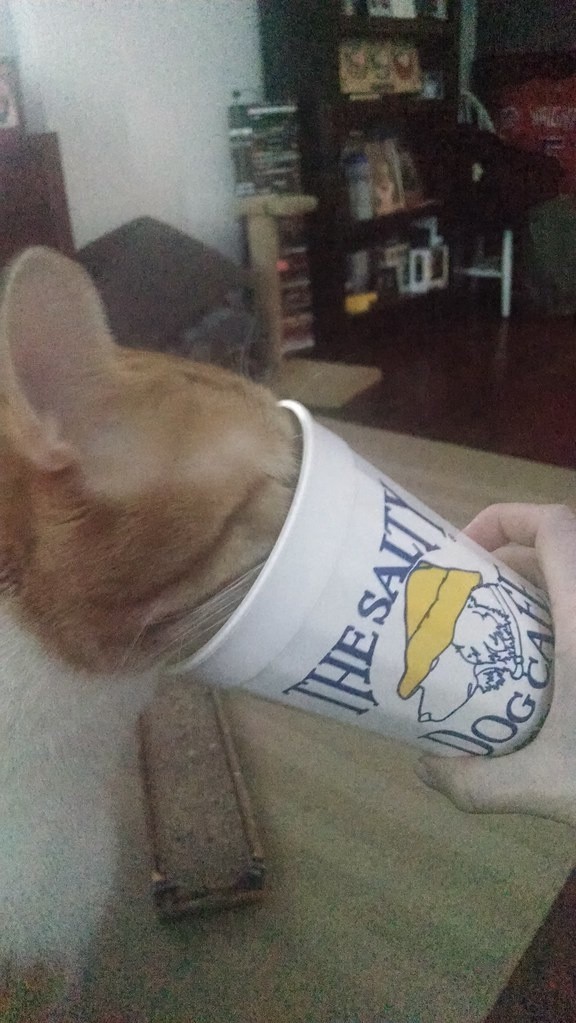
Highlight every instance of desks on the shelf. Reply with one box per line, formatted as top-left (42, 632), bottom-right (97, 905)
top-left (89, 411), bottom-right (576, 1023)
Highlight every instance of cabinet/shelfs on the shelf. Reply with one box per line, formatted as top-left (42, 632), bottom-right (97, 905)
top-left (256, 0), bottom-right (465, 369)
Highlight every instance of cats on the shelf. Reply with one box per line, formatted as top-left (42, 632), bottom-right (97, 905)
top-left (1, 242), bottom-right (304, 1023)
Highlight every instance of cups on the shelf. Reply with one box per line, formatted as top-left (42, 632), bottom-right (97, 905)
top-left (164, 400), bottom-right (556, 757)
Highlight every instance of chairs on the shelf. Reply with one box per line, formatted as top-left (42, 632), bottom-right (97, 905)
top-left (457, 88), bottom-right (563, 314)
